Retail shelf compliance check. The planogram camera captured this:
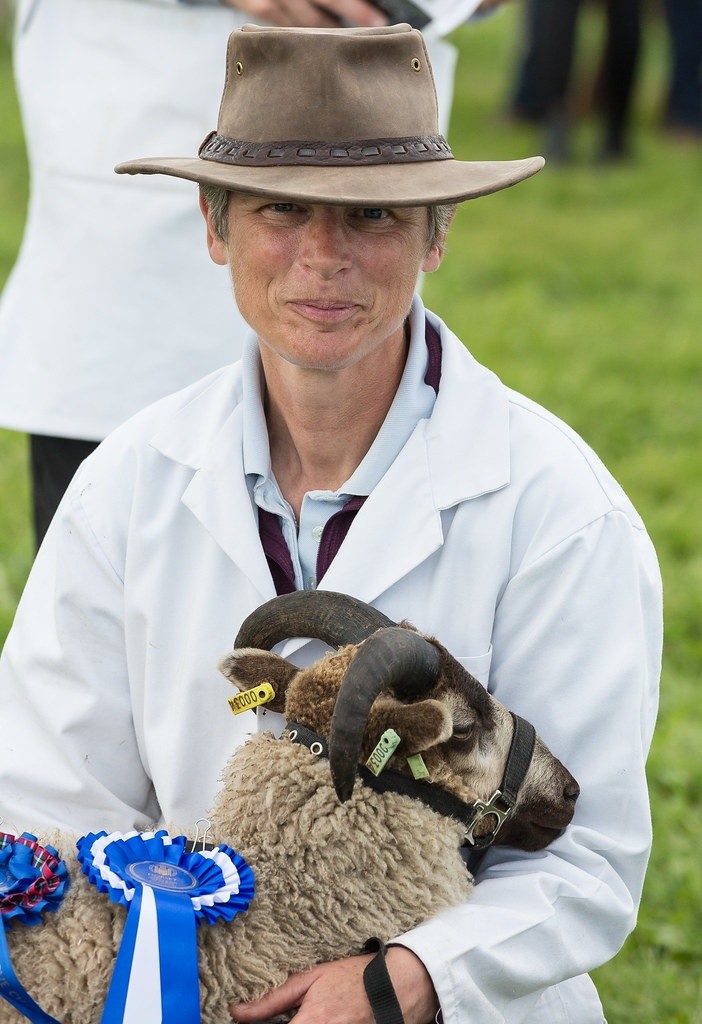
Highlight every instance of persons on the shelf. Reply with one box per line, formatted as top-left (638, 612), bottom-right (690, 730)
top-left (510, 1), bottom-right (700, 162)
top-left (1, 32), bottom-right (663, 1023)
top-left (1, 1), bottom-right (502, 562)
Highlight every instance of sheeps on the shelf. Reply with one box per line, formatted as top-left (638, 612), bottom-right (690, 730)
top-left (0, 586), bottom-right (583, 1024)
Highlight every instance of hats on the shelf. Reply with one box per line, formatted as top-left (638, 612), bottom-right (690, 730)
top-left (114, 23), bottom-right (544, 210)
